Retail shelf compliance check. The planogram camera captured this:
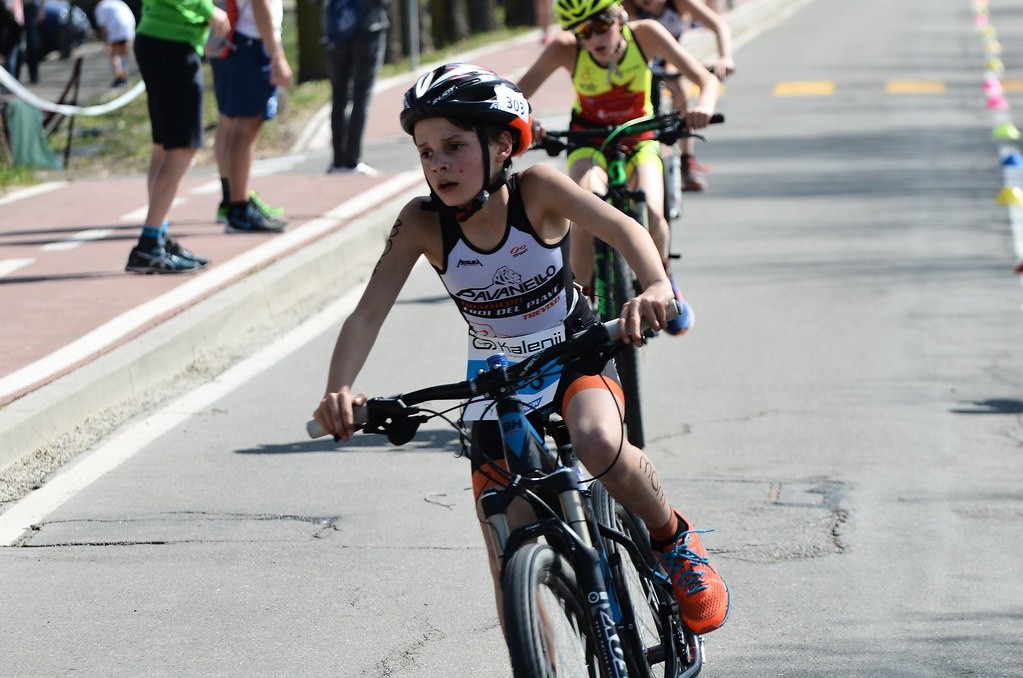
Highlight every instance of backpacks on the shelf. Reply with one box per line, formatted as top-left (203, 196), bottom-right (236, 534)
top-left (326, 0), bottom-right (367, 47)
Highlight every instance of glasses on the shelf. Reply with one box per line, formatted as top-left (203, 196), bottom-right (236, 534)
top-left (574, 15), bottom-right (613, 40)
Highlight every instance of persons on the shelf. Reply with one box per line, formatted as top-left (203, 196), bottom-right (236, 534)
top-left (0, 0), bottom-right (46, 93)
top-left (94, 0), bottom-right (135, 89)
top-left (516, 0), bottom-right (719, 338)
top-left (321, 0), bottom-right (391, 178)
top-left (538, 0), bottom-right (553, 46)
top-left (126, 0), bottom-right (231, 274)
top-left (205, 0), bottom-right (294, 234)
top-left (621, 0), bottom-right (734, 191)
top-left (314, 63), bottom-right (731, 678)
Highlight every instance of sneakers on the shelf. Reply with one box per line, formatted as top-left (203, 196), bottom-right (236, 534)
top-left (328, 163), bottom-right (377, 176)
top-left (225, 201), bottom-right (287, 232)
top-left (162, 233), bottom-right (213, 268)
top-left (125, 246), bottom-right (200, 274)
top-left (216, 193), bottom-right (284, 220)
top-left (664, 274), bottom-right (695, 336)
top-left (649, 509), bottom-right (731, 634)
top-left (681, 158), bottom-right (707, 190)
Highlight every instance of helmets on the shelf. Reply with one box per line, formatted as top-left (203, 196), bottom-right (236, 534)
top-left (556, 0), bottom-right (624, 30)
top-left (399, 64), bottom-right (532, 156)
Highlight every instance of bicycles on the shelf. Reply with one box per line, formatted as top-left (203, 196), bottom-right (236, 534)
top-left (645, 63), bottom-right (720, 260)
top-left (306, 301), bottom-right (706, 678)
top-left (526, 110), bottom-right (725, 452)
